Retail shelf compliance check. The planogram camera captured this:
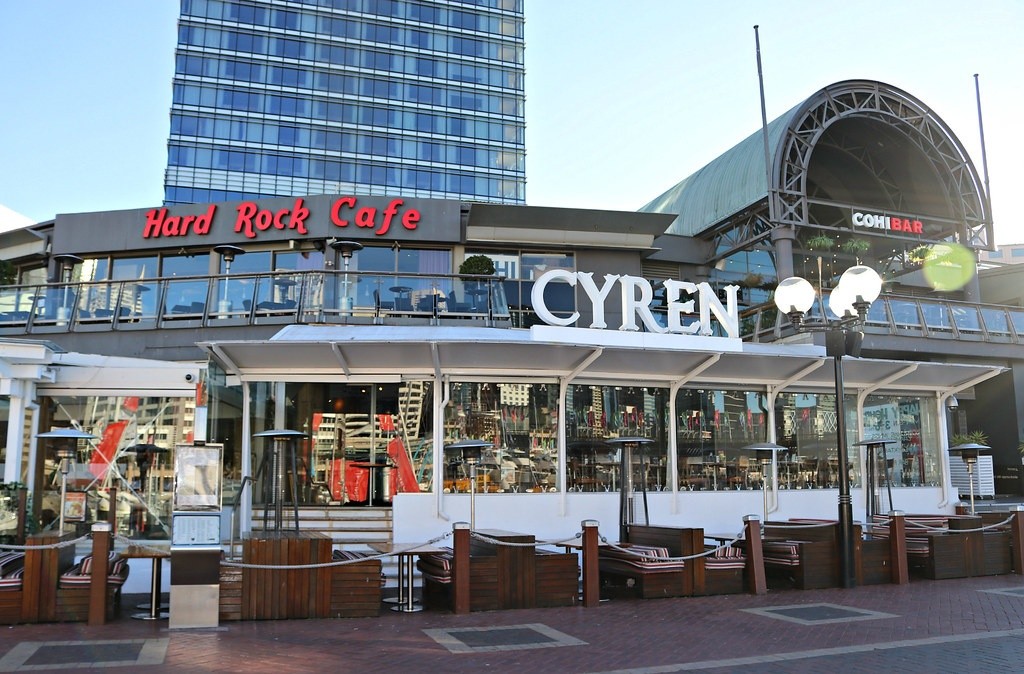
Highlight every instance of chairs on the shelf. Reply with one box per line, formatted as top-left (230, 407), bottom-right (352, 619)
top-left (243, 299), bottom-right (297, 317)
top-left (373, 290), bottom-right (395, 318)
top-left (170, 302), bottom-right (204, 321)
top-left (77, 307), bottom-right (131, 324)
top-left (418, 291), bottom-right (471, 320)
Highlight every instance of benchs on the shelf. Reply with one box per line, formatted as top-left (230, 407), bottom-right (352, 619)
top-left (418, 529), bottom-right (579, 612)
top-left (763, 518), bottom-right (893, 590)
top-left (220, 529), bottom-right (384, 620)
top-left (0, 529), bottom-right (129, 624)
top-left (598, 524), bottom-right (750, 599)
top-left (872, 511), bottom-right (1023, 580)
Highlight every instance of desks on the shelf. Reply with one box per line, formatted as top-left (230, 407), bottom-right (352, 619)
top-left (467, 290), bottom-right (487, 308)
top-left (368, 542), bottom-right (448, 613)
top-left (863, 527), bottom-right (932, 538)
top-left (122, 543), bottom-right (171, 621)
top-left (388, 286), bottom-right (412, 298)
top-left (535, 535), bottom-right (631, 554)
top-left (272, 279), bottom-right (295, 316)
top-left (704, 535), bottom-right (788, 548)
top-left (3, 311), bottom-right (39, 321)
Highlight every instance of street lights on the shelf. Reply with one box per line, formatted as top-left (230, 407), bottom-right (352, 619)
top-left (774, 265), bottom-right (881, 588)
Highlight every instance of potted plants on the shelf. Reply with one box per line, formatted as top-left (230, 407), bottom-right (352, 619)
top-left (458, 254), bottom-right (497, 313)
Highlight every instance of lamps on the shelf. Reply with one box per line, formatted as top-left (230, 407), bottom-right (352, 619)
top-left (212, 245), bottom-right (246, 318)
top-left (331, 241), bottom-right (364, 317)
top-left (53, 253), bottom-right (84, 326)
